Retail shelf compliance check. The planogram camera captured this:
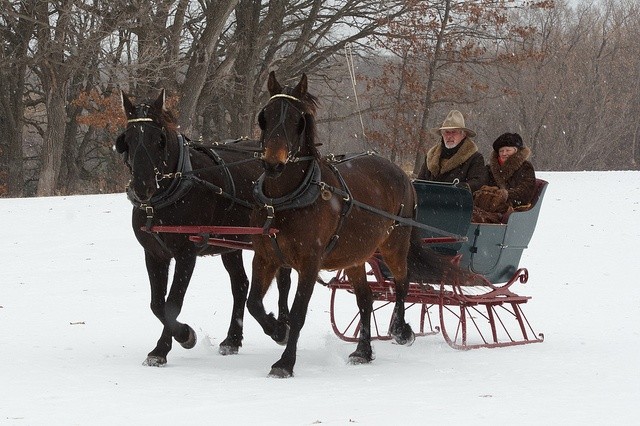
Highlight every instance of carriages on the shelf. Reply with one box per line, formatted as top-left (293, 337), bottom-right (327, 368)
top-left (110, 70), bottom-right (546, 378)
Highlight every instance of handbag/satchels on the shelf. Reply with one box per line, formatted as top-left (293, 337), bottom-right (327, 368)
top-left (474, 185), bottom-right (505, 212)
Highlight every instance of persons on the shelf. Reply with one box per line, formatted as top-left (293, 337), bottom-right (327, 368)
top-left (417, 110), bottom-right (485, 197)
top-left (482, 132), bottom-right (536, 224)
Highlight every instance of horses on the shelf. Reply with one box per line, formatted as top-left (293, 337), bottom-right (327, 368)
top-left (246, 70), bottom-right (489, 380)
top-left (114, 87), bottom-right (293, 367)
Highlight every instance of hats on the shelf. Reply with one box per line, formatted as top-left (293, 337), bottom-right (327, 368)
top-left (429, 109), bottom-right (476, 137)
top-left (492, 132), bottom-right (522, 152)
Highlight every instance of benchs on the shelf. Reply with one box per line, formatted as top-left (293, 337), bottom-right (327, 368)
top-left (495, 176), bottom-right (544, 224)
